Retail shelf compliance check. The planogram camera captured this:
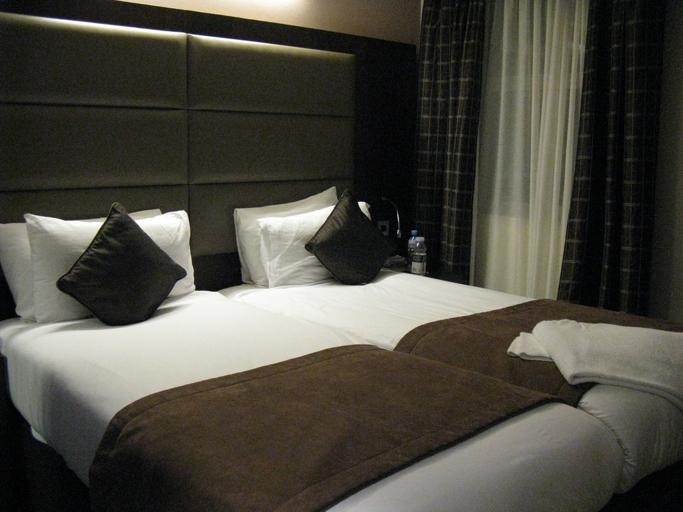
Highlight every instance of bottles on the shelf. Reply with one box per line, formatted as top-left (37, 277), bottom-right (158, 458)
top-left (407, 230), bottom-right (427, 277)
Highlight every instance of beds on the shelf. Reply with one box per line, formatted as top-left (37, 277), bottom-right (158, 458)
top-left (217, 269), bottom-right (683, 495)
top-left (0, 292), bottom-right (624, 510)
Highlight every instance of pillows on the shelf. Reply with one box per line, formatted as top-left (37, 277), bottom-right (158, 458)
top-left (2, 222), bottom-right (34, 322)
top-left (55, 201), bottom-right (189, 328)
top-left (23, 208), bottom-right (195, 324)
top-left (258, 201), bottom-right (372, 289)
top-left (232, 185), bottom-right (339, 285)
top-left (303, 187), bottom-right (395, 288)
